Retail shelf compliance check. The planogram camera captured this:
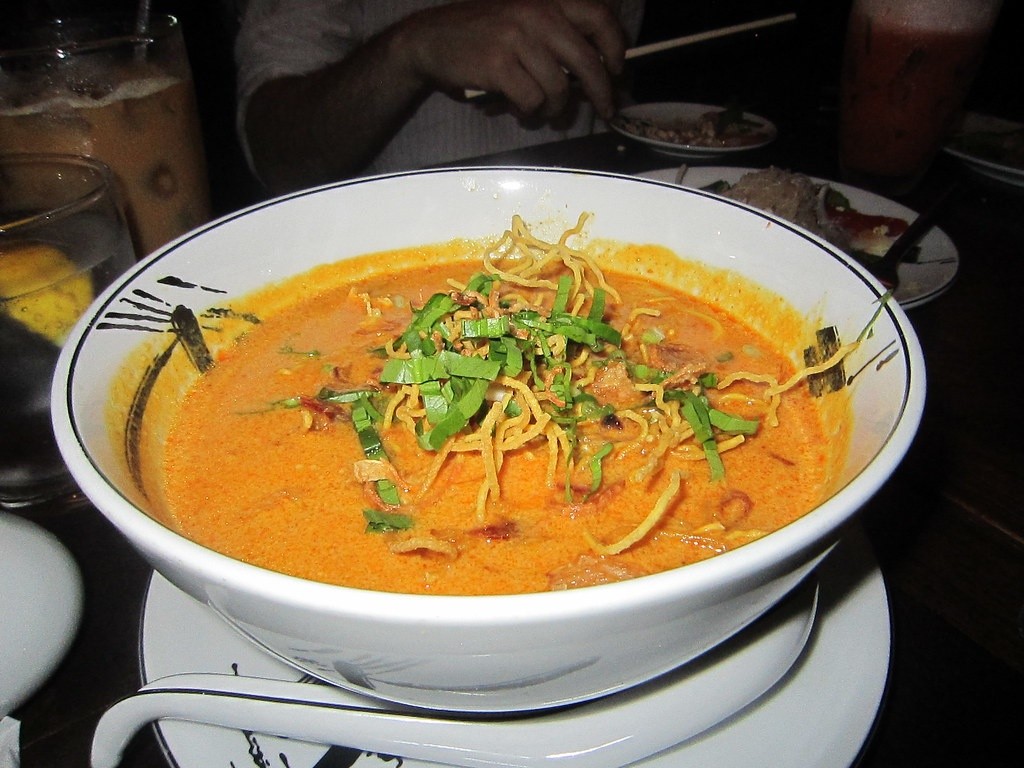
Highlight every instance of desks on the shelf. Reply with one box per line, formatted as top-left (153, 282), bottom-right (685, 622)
top-left (3, 104), bottom-right (1024, 768)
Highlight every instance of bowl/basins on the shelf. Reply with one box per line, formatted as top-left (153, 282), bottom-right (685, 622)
top-left (48, 162), bottom-right (927, 714)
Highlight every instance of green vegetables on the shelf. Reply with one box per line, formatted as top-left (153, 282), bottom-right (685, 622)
top-left (264, 270), bottom-right (759, 531)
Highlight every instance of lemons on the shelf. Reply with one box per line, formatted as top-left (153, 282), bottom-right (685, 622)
top-left (0, 243), bottom-right (99, 348)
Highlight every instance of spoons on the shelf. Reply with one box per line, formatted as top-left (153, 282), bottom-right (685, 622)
top-left (89, 584), bottom-right (822, 768)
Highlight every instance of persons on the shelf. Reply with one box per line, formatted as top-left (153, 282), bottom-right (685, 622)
top-left (235, 0), bottom-right (640, 193)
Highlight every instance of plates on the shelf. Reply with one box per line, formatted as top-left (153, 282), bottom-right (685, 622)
top-left (0, 510), bottom-right (83, 718)
top-left (633, 165), bottom-right (961, 311)
top-left (135, 568), bottom-right (891, 768)
top-left (942, 145), bottom-right (1024, 188)
top-left (608, 102), bottom-right (778, 159)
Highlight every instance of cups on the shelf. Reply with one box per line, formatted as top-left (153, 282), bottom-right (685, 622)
top-left (840, 0), bottom-right (1000, 172)
top-left (0, 11), bottom-right (216, 260)
top-left (0, 148), bottom-right (139, 519)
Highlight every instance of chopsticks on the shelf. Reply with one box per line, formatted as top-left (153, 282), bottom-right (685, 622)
top-left (464, 13), bottom-right (797, 100)
